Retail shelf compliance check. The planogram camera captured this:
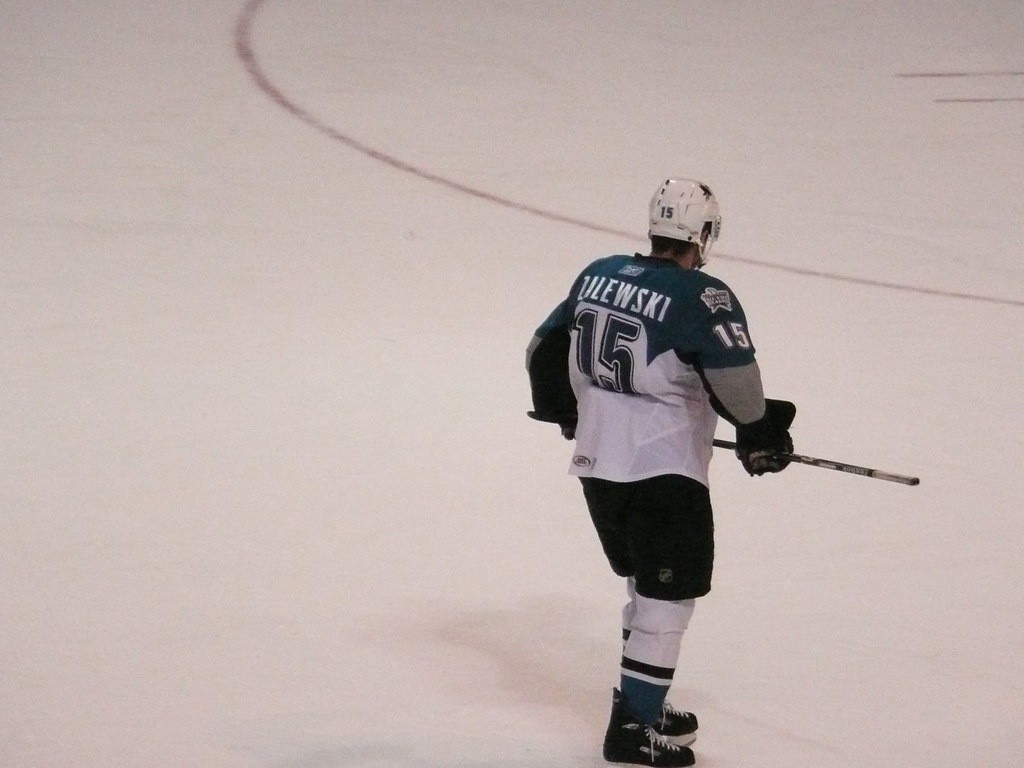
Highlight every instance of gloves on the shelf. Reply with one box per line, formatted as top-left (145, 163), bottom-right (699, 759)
top-left (736, 399), bottom-right (798, 475)
top-left (559, 419), bottom-right (578, 441)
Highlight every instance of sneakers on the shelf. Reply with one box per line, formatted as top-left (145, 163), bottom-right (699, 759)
top-left (601, 685), bottom-right (696, 768)
top-left (619, 682), bottom-right (698, 748)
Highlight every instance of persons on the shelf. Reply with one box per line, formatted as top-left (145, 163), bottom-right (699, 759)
top-left (527, 176), bottom-right (796, 768)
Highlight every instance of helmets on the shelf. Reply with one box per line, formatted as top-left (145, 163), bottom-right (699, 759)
top-left (648, 178), bottom-right (720, 264)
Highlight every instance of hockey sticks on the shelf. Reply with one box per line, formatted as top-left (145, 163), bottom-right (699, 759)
top-left (526, 410), bottom-right (919, 486)
